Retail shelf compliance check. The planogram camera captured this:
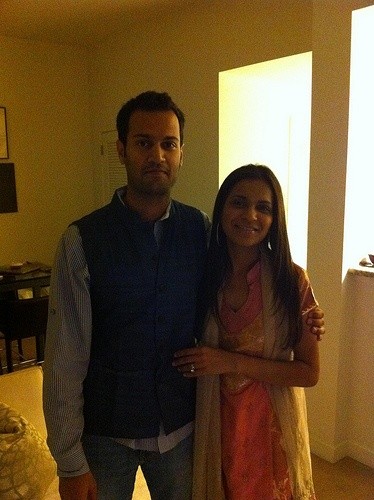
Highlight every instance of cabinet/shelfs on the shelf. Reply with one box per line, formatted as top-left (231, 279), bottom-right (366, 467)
top-left (0, 260), bottom-right (53, 375)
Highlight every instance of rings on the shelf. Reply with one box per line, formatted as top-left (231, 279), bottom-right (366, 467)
top-left (190, 363), bottom-right (195, 373)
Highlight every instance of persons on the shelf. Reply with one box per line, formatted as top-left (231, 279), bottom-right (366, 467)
top-left (172, 163), bottom-right (320, 500)
top-left (42, 90), bottom-right (326, 500)
